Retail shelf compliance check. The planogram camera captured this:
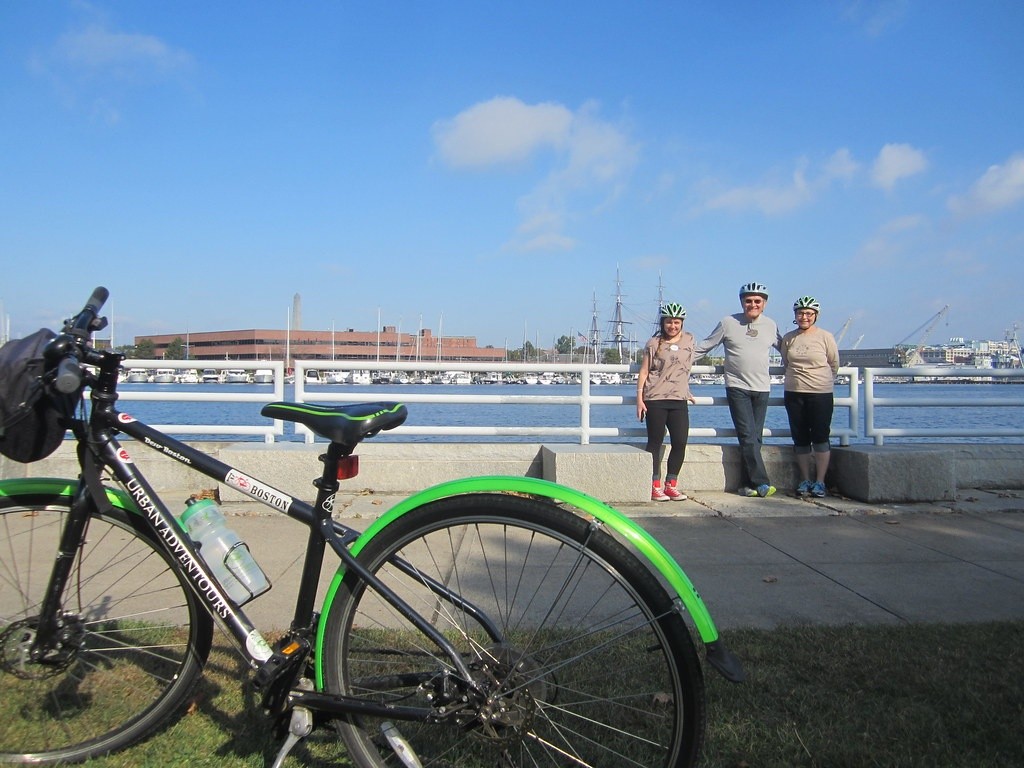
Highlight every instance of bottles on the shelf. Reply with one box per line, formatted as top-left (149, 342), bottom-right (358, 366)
top-left (180, 497), bottom-right (270, 606)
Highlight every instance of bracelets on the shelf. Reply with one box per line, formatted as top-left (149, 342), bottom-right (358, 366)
top-left (637, 389), bottom-right (642, 391)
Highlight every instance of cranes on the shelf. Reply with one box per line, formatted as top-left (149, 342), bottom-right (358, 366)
top-left (831, 316), bottom-right (853, 350)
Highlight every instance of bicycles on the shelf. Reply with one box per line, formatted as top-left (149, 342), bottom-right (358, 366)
top-left (1, 285), bottom-right (749, 768)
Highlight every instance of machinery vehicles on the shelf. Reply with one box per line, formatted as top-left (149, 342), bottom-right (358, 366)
top-left (887, 303), bottom-right (950, 368)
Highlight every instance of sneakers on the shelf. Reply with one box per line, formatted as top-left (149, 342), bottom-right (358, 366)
top-left (796, 479), bottom-right (812, 497)
top-left (664, 479), bottom-right (687, 500)
top-left (738, 485), bottom-right (758, 497)
top-left (652, 480), bottom-right (670, 501)
top-left (758, 484), bottom-right (776, 497)
top-left (812, 481), bottom-right (826, 498)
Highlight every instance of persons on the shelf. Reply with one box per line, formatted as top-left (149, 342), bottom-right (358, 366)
top-left (694, 283), bottom-right (783, 498)
top-left (781, 296), bottom-right (839, 499)
top-left (636, 303), bottom-right (697, 501)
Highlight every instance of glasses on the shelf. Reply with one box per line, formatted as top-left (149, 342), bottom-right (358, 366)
top-left (744, 299), bottom-right (764, 304)
top-left (796, 311), bottom-right (816, 317)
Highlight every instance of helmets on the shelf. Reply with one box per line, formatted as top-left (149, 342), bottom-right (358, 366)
top-left (793, 297), bottom-right (819, 313)
top-left (740, 283), bottom-right (769, 298)
top-left (660, 302), bottom-right (686, 320)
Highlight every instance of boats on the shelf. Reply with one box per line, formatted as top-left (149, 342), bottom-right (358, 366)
top-left (77, 255), bottom-right (1024, 387)
